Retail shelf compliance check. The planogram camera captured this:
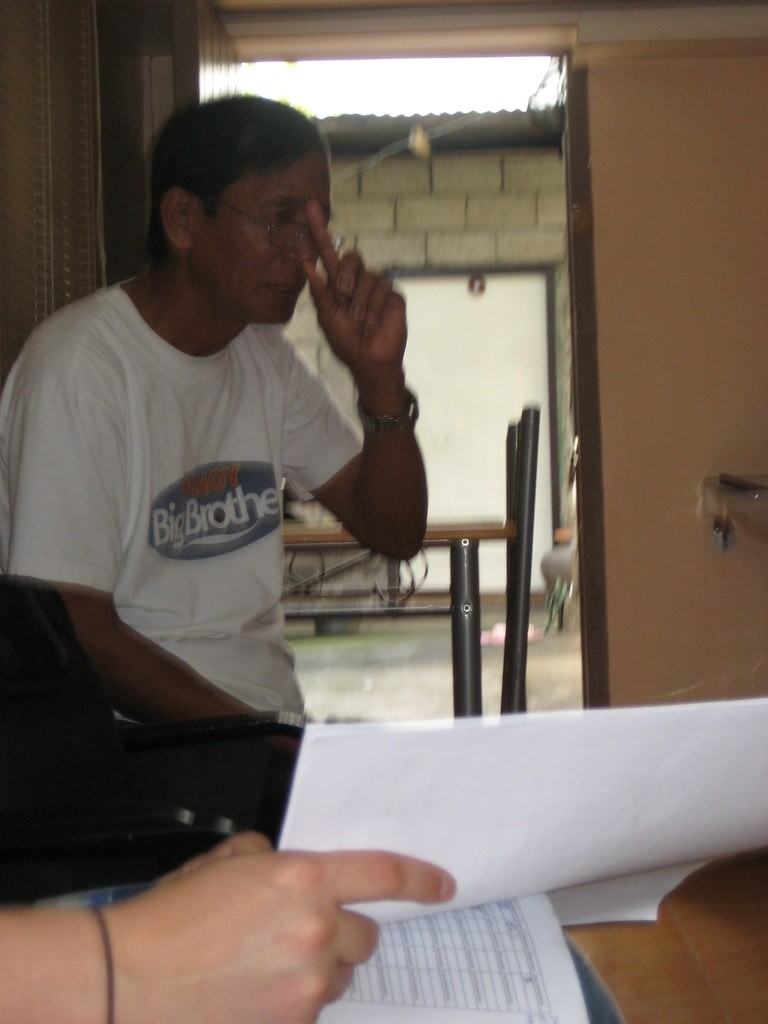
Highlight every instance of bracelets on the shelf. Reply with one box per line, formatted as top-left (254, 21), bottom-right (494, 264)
top-left (356, 384), bottom-right (421, 432)
top-left (89, 905), bottom-right (114, 1024)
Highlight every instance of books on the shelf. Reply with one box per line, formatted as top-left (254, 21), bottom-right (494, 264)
top-left (317, 890), bottom-right (591, 1024)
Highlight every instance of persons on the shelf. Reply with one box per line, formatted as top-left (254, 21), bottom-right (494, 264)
top-left (0, 829), bottom-right (456, 1024)
top-left (0, 95), bottom-right (427, 728)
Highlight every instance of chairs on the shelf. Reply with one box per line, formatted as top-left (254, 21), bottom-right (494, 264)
top-left (0, 571), bottom-right (307, 910)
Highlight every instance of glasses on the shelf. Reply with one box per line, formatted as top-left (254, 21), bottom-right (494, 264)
top-left (204, 190), bottom-right (319, 249)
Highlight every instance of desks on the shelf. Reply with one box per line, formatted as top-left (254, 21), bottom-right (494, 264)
top-left (277, 517), bottom-right (519, 722)
